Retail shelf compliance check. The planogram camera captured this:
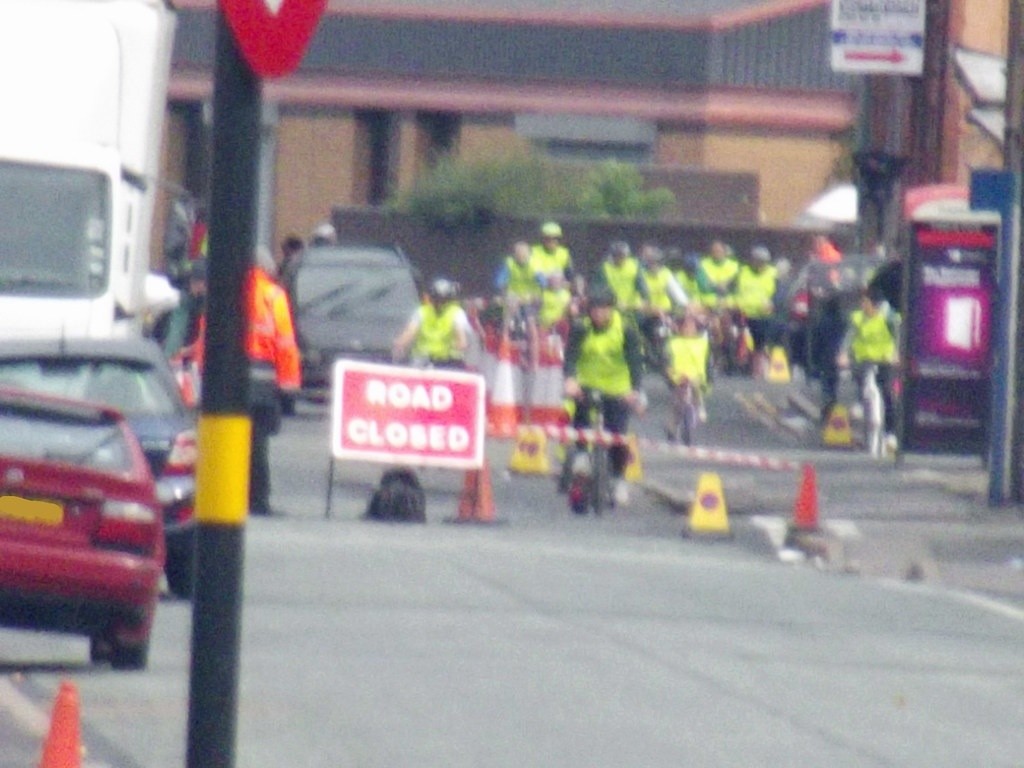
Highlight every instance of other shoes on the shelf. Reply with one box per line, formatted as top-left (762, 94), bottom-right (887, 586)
top-left (611, 477), bottom-right (630, 506)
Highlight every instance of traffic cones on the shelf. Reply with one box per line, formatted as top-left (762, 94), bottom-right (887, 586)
top-left (447, 443), bottom-right (506, 526)
top-left (473, 316), bottom-right (575, 440)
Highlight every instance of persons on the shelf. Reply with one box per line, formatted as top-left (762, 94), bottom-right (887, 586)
top-left (389, 218), bottom-right (901, 516)
top-left (195, 243), bottom-right (302, 520)
top-left (277, 223), bottom-right (338, 278)
top-left (849, 136), bottom-right (912, 256)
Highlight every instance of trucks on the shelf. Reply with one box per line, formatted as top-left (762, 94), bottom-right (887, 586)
top-left (0, 0), bottom-right (182, 348)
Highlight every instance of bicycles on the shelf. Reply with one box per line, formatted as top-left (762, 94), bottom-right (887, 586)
top-left (665, 369), bottom-right (711, 454)
top-left (840, 348), bottom-right (909, 468)
top-left (401, 259), bottom-right (781, 412)
top-left (560, 381), bottom-right (641, 513)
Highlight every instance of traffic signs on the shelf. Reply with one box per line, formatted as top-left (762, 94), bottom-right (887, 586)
top-left (825, 0), bottom-right (927, 76)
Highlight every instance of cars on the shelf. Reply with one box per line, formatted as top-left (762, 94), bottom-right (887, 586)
top-left (0, 338), bottom-right (200, 604)
top-left (781, 249), bottom-right (868, 365)
top-left (1, 382), bottom-right (166, 671)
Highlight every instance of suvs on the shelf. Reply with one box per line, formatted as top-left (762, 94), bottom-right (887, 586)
top-left (271, 242), bottom-right (433, 419)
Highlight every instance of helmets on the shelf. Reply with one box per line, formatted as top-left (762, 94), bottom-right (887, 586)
top-left (432, 278), bottom-right (453, 298)
top-left (751, 244), bottom-right (771, 262)
top-left (540, 219), bottom-right (563, 239)
top-left (610, 239), bottom-right (631, 255)
top-left (586, 280), bottom-right (617, 309)
